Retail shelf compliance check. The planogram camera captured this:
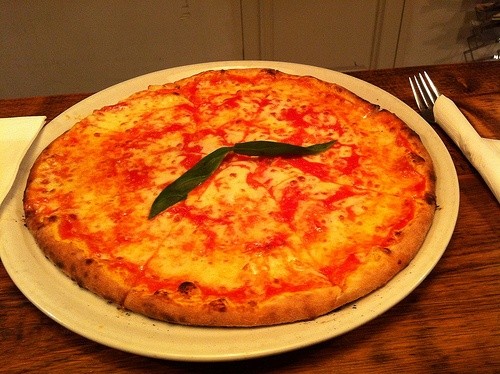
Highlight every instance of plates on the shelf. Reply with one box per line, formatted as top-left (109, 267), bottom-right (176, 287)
top-left (0, 60), bottom-right (460, 361)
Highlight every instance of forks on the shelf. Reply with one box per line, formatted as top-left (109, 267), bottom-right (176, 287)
top-left (409, 71), bottom-right (442, 124)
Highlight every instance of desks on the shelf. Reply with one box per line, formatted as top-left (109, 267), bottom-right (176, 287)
top-left (0, 61), bottom-right (500, 374)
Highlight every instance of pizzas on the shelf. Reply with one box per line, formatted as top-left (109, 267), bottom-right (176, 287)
top-left (22, 67), bottom-right (436, 328)
